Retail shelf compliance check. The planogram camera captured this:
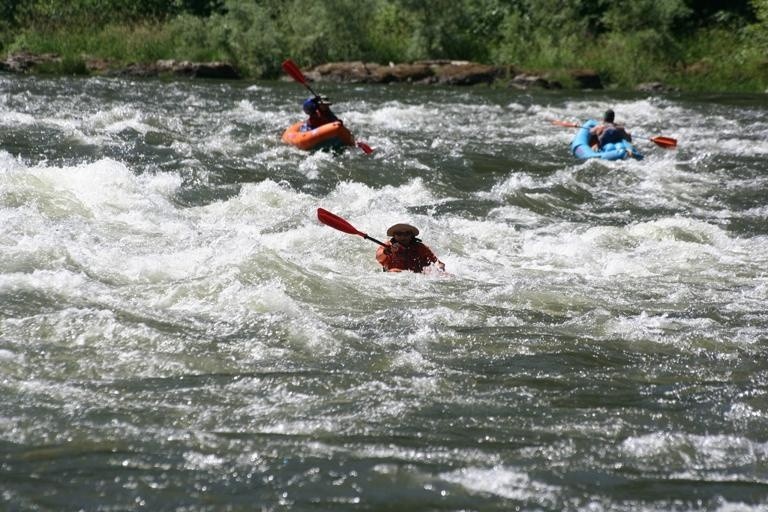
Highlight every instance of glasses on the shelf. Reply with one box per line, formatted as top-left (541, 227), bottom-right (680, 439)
top-left (395, 230), bottom-right (410, 238)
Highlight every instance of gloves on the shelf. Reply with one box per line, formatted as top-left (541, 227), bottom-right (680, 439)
top-left (311, 97), bottom-right (321, 103)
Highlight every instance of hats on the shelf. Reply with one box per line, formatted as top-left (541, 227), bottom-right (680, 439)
top-left (318, 92), bottom-right (333, 105)
top-left (604, 109), bottom-right (614, 121)
top-left (386, 224), bottom-right (419, 238)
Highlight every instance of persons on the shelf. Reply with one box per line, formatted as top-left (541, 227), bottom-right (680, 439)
top-left (299, 94), bottom-right (336, 132)
top-left (376, 222), bottom-right (446, 273)
top-left (588, 108), bottom-right (619, 153)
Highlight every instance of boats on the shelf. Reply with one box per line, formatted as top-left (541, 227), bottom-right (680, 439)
top-left (570, 116), bottom-right (644, 161)
top-left (388, 267), bottom-right (455, 282)
top-left (279, 120), bottom-right (357, 153)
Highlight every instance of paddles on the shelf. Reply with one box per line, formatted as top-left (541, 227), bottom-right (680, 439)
top-left (552, 119), bottom-right (677, 150)
top-left (281, 58), bottom-right (371, 155)
top-left (317, 208), bottom-right (390, 249)
